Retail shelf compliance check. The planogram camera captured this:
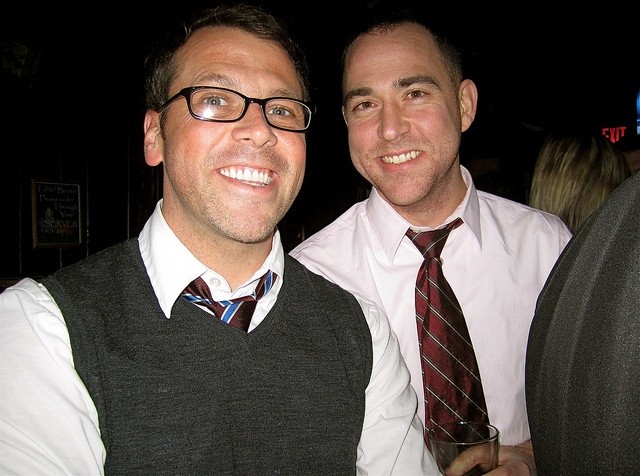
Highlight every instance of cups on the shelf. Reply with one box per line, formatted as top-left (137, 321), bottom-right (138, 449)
top-left (427, 421), bottom-right (500, 476)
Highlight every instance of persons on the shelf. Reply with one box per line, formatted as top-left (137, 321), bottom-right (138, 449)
top-left (287, 11), bottom-right (578, 475)
top-left (526, 126), bottom-right (631, 236)
top-left (1, 4), bottom-right (441, 475)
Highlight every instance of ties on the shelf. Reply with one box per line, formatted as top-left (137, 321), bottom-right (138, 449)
top-left (181, 269), bottom-right (277, 332)
top-left (405, 218), bottom-right (494, 472)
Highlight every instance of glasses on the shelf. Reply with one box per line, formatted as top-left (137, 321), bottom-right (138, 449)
top-left (154, 85), bottom-right (318, 132)
